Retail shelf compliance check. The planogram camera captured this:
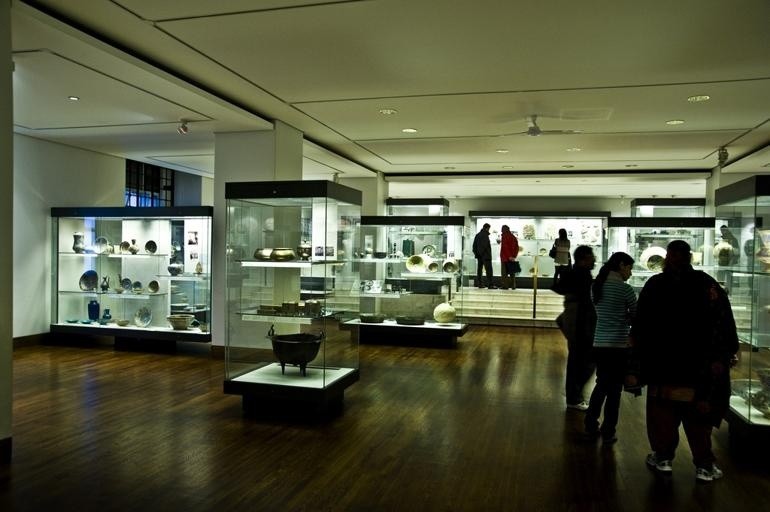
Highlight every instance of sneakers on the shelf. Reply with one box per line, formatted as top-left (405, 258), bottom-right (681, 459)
top-left (567, 401), bottom-right (589, 411)
top-left (647, 453), bottom-right (673, 471)
top-left (590, 426), bottom-right (618, 443)
top-left (697, 464), bottom-right (723, 482)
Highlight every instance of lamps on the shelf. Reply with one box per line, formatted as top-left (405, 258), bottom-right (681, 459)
top-left (176, 125), bottom-right (188, 137)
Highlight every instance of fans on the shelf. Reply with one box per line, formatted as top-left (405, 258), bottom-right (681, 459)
top-left (505, 114), bottom-right (573, 137)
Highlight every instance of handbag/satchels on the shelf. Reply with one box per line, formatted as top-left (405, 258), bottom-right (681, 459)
top-left (556, 309), bottom-right (589, 341)
top-left (549, 246), bottom-right (556, 258)
top-left (505, 260), bottom-right (521, 274)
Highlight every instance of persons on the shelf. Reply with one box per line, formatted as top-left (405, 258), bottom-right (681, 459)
top-left (583, 251), bottom-right (639, 444)
top-left (472, 223), bottom-right (499, 290)
top-left (564, 423), bottom-right (618, 510)
top-left (624, 240), bottom-right (741, 483)
top-left (561, 404), bottom-right (587, 434)
top-left (551, 228), bottom-right (571, 285)
top-left (499, 225), bottom-right (520, 290)
top-left (555, 245), bottom-right (598, 412)
top-left (645, 460), bottom-right (719, 511)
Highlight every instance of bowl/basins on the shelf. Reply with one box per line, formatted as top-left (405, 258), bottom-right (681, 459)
top-left (167, 314), bottom-right (195, 330)
top-left (113, 288), bottom-right (145, 294)
top-left (118, 320), bottom-right (129, 326)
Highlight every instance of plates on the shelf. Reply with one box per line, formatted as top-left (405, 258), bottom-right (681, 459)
top-left (144, 240), bottom-right (157, 254)
top-left (134, 306), bottom-right (152, 327)
top-left (121, 278), bottom-right (159, 293)
top-left (78, 270), bottom-right (98, 291)
